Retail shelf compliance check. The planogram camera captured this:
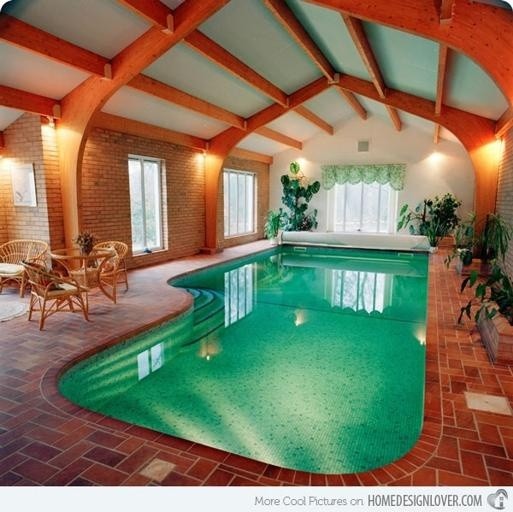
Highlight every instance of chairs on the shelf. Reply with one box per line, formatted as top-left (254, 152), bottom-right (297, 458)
top-left (19, 261), bottom-right (91, 332)
top-left (0, 239), bottom-right (49, 299)
top-left (84, 241), bottom-right (128, 304)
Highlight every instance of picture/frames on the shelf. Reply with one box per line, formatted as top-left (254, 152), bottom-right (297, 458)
top-left (11, 162), bottom-right (38, 207)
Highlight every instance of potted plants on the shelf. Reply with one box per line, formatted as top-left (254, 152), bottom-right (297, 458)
top-left (73, 230), bottom-right (98, 256)
top-left (421, 212), bottom-right (513, 276)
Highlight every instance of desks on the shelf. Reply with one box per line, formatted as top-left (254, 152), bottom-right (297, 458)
top-left (48, 248), bottom-right (118, 314)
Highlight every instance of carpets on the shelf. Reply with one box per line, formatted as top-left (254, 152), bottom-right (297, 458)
top-left (0, 288), bottom-right (34, 323)
